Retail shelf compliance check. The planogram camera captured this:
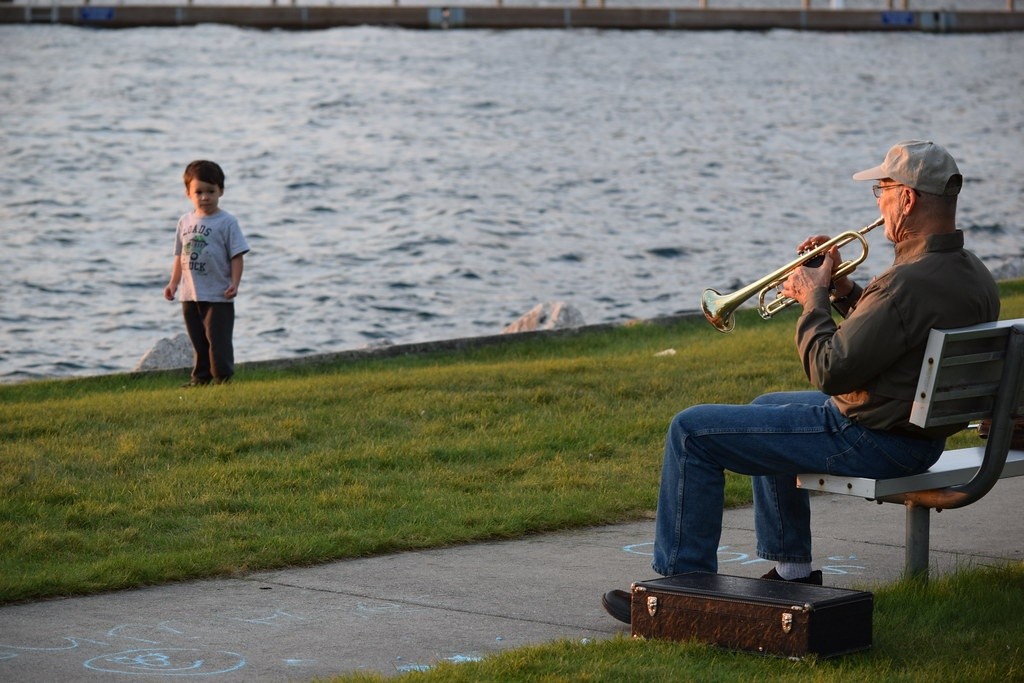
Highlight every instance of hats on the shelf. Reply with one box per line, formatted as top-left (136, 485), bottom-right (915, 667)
top-left (853, 140), bottom-right (963, 196)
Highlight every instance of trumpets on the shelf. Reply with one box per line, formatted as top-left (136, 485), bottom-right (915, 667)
top-left (699, 211), bottom-right (884, 335)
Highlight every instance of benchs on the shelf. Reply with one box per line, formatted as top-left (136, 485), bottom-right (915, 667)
top-left (796, 317), bottom-right (1024, 591)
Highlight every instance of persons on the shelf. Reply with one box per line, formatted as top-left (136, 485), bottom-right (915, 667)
top-left (163, 160), bottom-right (250, 388)
top-left (601, 140), bottom-right (1001, 625)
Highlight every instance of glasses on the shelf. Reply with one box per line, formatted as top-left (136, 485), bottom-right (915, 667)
top-left (872, 183), bottom-right (922, 199)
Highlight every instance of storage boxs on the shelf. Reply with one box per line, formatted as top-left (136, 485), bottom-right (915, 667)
top-left (632, 571), bottom-right (874, 661)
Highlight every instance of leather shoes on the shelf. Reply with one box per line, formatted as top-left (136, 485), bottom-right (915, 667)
top-left (602, 589), bottom-right (631, 624)
top-left (758, 569), bottom-right (824, 589)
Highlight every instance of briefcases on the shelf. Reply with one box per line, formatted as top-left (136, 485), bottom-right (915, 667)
top-left (631, 570), bottom-right (875, 661)
